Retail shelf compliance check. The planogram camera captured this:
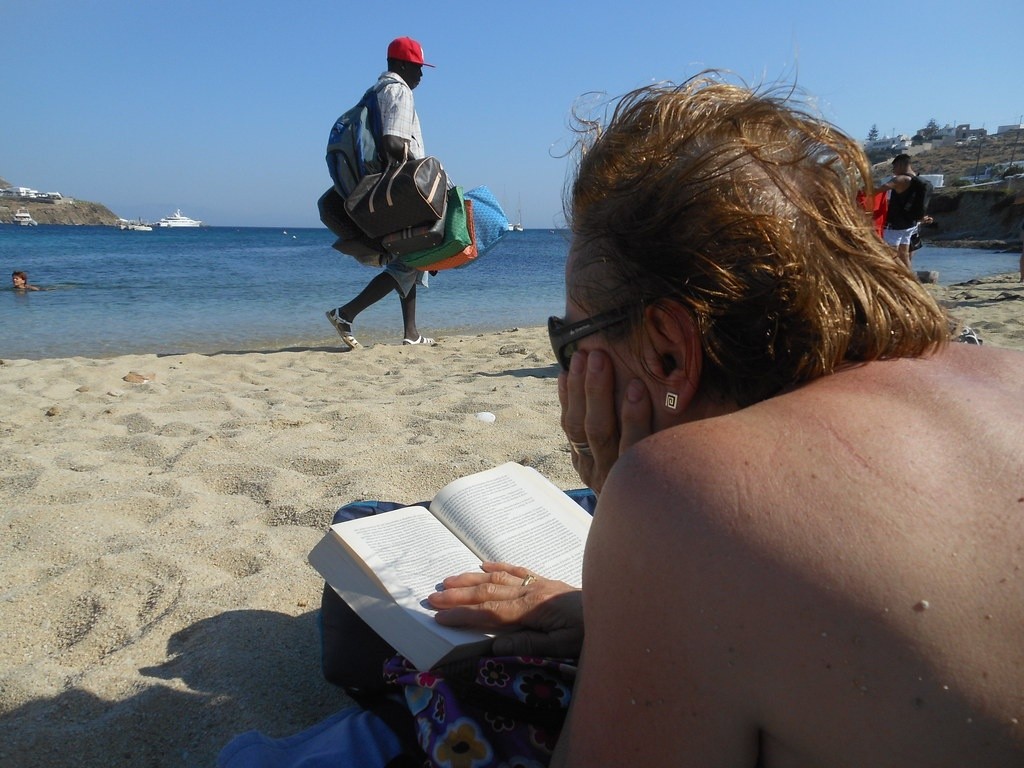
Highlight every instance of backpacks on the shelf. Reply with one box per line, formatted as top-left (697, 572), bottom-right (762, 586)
top-left (325, 80), bottom-right (416, 202)
top-left (900, 172), bottom-right (934, 222)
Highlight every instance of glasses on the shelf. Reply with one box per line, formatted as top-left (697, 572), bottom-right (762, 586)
top-left (547, 299), bottom-right (650, 374)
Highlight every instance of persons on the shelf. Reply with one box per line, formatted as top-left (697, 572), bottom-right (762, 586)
top-left (430, 64), bottom-right (1023, 767)
top-left (325, 35), bottom-right (438, 349)
top-left (11, 271), bottom-right (39, 292)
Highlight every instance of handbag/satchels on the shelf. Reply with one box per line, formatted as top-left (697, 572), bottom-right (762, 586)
top-left (319, 487), bottom-right (601, 768)
top-left (318, 141), bottom-right (509, 272)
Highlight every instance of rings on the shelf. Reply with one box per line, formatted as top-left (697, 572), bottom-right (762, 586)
top-left (522, 574), bottom-right (534, 585)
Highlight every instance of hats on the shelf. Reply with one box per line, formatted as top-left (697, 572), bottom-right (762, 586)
top-left (388, 36), bottom-right (436, 68)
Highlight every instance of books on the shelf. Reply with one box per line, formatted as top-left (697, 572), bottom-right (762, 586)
top-left (305, 460), bottom-right (593, 674)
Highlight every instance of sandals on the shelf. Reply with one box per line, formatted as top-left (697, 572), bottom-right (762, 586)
top-left (402, 335), bottom-right (436, 345)
top-left (326, 308), bottom-right (366, 350)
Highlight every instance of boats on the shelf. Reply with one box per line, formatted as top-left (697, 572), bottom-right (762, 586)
top-left (12, 207), bottom-right (32, 226)
top-left (115, 218), bottom-right (152, 231)
top-left (158, 209), bottom-right (202, 227)
top-left (508, 223), bottom-right (524, 232)
